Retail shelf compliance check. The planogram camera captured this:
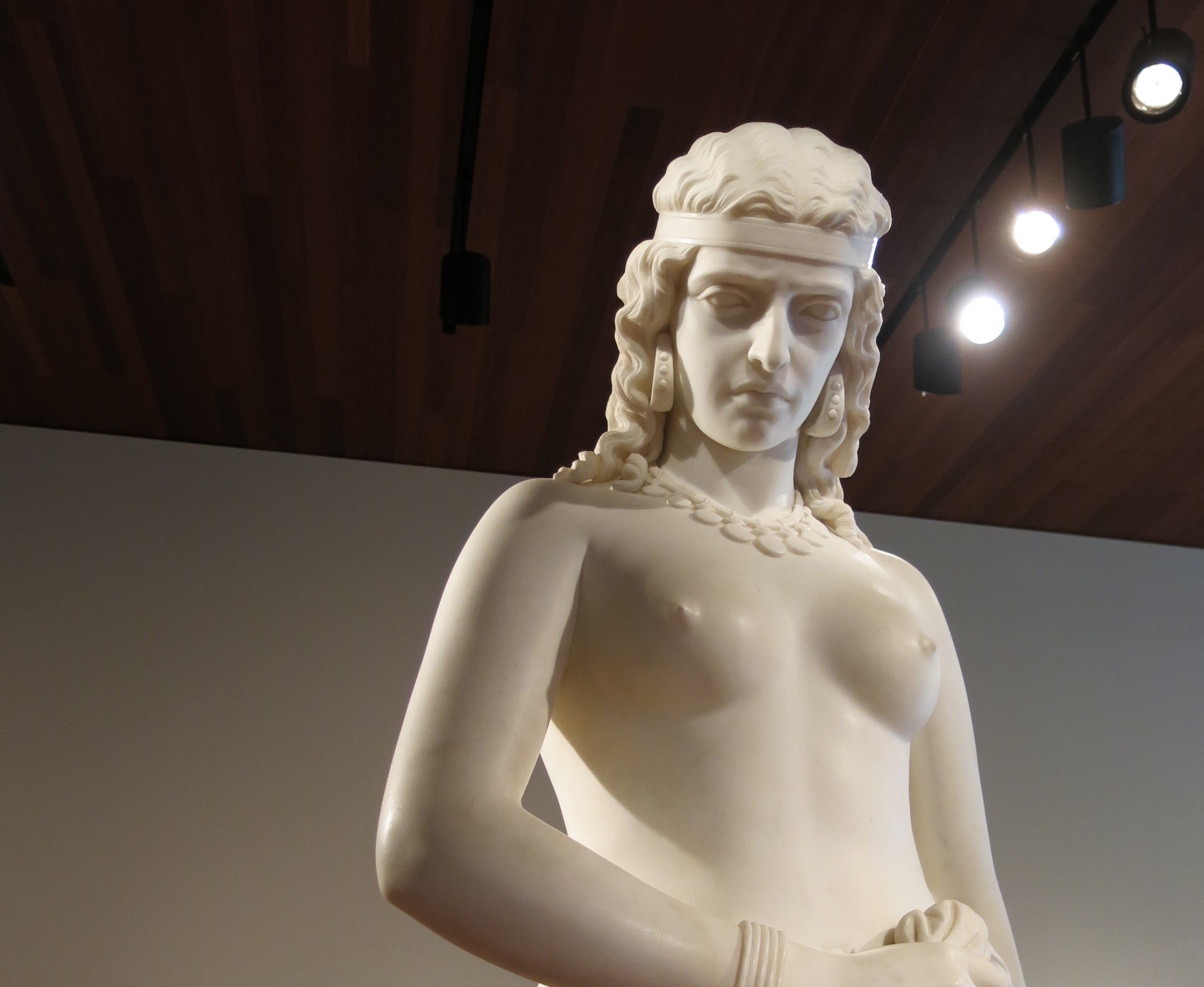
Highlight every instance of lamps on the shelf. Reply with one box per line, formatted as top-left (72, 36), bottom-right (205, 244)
top-left (911, 281), bottom-right (967, 399)
top-left (958, 215), bottom-right (1006, 345)
top-left (1008, 148), bottom-right (1060, 255)
top-left (1119, 1), bottom-right (1192, 123)
top-left (1059, 51), bottom-right (1129, 212)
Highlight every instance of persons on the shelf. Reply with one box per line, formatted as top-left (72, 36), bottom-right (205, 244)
top-left (368, 118), bottom-right (1029, 987)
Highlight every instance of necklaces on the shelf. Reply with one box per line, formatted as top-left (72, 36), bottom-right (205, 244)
top-left (636, 455), bottom-right (831, 560)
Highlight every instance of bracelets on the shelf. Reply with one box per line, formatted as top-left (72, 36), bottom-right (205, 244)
top-left (733, 921), bottom-right (786, 987)
